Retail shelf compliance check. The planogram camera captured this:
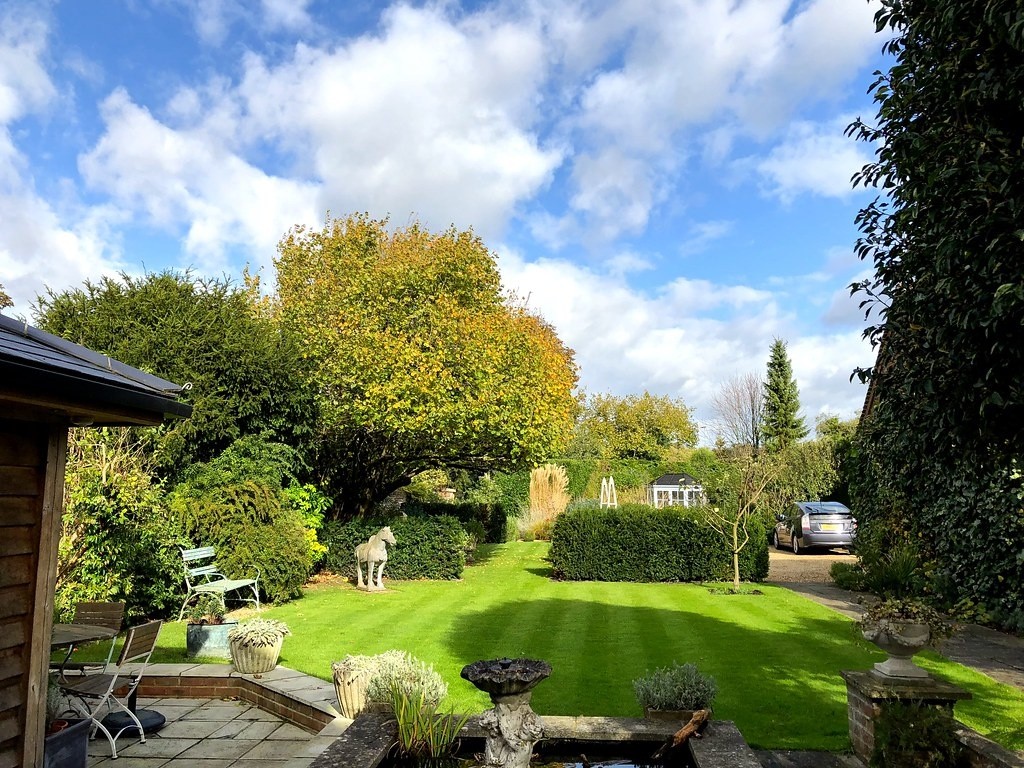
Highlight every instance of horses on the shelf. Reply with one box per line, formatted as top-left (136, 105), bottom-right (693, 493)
top-left (354, 526), bottom-right (397, 591)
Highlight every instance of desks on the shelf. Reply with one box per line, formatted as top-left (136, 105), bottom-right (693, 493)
top-left (50, 622), bottom-right (117, 685)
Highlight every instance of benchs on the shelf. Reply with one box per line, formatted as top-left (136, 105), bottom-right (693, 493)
top-left (177, 545), bottom-right (261, 622)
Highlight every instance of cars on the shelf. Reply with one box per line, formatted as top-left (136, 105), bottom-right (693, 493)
top-left (768, 501), bottom-right (859, 555)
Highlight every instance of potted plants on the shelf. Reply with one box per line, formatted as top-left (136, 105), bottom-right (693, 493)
top-left (852, 598), bottom-right (955, 677)
top-left (187, 592), bottom-right (239, 661)
top-left (44, 685), bottom-right (92, 768)
top-left (365, 647), bottom-right (449, 720)
top-left (631, 659), bottom-right (717, 720)
top-left (330, 649), bottom-right (397, 720)
top-left (227, 615), bottom-right (292, 674)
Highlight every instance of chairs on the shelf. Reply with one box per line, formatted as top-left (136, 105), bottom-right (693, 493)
top-left (49, 601), bottom-right (124, 675)
top-left (58, 620), bottom-right (164, 759)
